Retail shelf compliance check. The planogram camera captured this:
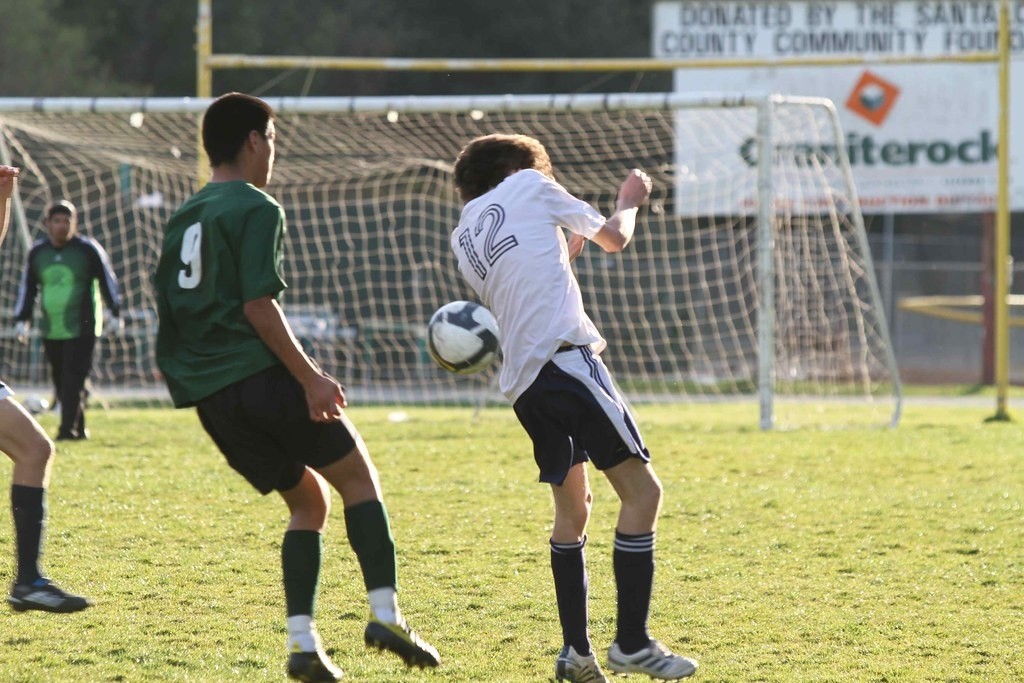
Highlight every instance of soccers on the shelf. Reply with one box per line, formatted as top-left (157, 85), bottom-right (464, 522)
top-left (426, 299), bottom-right (504, 377)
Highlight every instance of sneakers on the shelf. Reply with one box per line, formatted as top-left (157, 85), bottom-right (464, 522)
top-left (364, 609), bottom-right (441, 669)
top-left (9, 577), bottom-right (88, 613)
top-left (556, 645), bottom-right (608, 683)
top-left (287, 641), bottom-right (343, 682)
top-left (607, 637), bottom-right (698, 682)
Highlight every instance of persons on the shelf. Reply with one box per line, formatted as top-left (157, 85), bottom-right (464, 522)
top-left (448, 132), bottom-right (697, 683)
top-left (16, 200), bottom-right (123, 443)
top-left (154, 93), bottom-right (438, 683)
top-left (0, 164), bottom-right (89, 614)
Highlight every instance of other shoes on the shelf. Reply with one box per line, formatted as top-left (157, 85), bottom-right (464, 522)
top-left (57, 429), bottom-right (87, 440)
